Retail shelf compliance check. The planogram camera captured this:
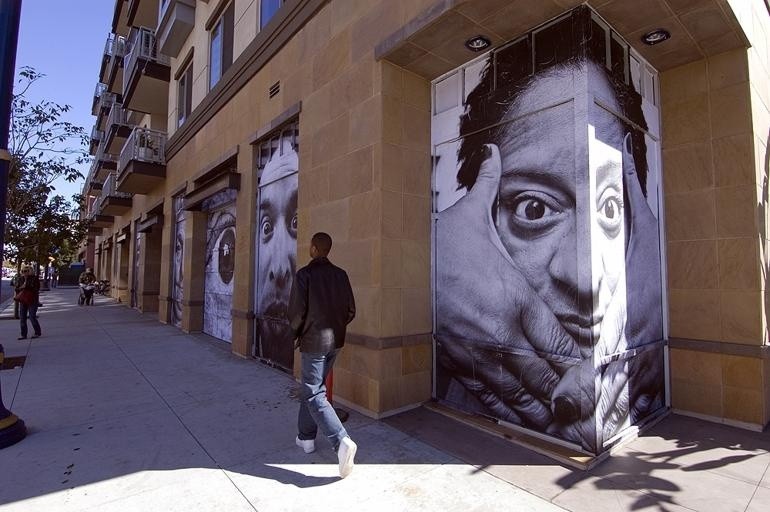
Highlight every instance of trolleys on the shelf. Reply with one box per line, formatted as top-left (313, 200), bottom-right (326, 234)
top-left (77, 284), bottom-right (95, 306)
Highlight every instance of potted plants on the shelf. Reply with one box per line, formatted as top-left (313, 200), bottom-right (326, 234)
top-left (132, 134), bottom-right (157, 161)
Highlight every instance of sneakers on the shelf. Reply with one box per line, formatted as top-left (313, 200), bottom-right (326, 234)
top-left (337, 436), bottom-right (357, 478)
top-left (295, 434), bottom-right (315, 454)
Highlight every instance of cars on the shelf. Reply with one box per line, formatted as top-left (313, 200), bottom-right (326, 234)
top-left (2, 267), bottom-right (17, 278)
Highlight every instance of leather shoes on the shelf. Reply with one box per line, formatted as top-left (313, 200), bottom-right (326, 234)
top-left (18, 336), bottom-right (27, 340)
top-left (31, 334), bottom-right (39, 338)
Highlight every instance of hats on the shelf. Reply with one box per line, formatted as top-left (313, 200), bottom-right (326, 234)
top-left (86, 267), bottom-right (92, 273)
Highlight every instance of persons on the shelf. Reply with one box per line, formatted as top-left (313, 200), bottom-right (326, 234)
top-left (173, 209), bottom-right (186, 320)
top-left (287, 232), bottom-right (357, 478)
top-left (255, 139), bottom-right (299, 370)
top-left (15, 266), bottom-right (41, 340)
top-left (431, 6), bottom-right (665, 451)
top-left (202, 200), bottom-right (236, 344)
top-left (51, 271), bottom-right (59, 288)
top-left (78, 267), bottom-right (96, 305)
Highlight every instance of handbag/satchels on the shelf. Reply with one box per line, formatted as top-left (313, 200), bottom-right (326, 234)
top-left (14, 289), bottom-right (36, 306)
